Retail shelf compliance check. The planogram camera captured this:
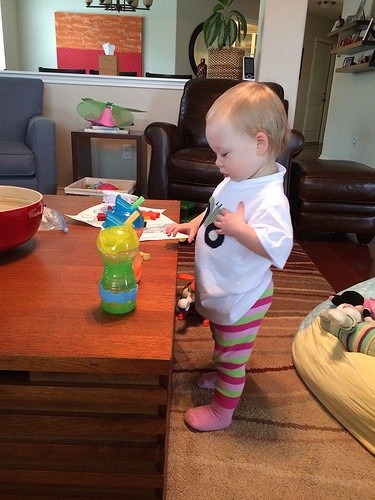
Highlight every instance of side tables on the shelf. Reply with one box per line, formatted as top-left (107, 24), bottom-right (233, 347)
top-left (70, 128), bottom-right (149, 190)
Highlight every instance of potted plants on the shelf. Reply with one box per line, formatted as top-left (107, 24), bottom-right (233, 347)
top-left (207, 0), bottom-right (249, 80)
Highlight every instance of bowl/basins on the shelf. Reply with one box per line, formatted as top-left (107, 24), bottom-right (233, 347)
top-left (0, 185), bottom-right (42, 253)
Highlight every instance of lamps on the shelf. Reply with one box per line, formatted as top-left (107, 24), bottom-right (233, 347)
top-left (84, 0), bottom-right (153, 13)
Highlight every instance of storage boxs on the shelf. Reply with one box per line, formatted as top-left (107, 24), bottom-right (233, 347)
top-left (64, 176), bottom-right (136, 196)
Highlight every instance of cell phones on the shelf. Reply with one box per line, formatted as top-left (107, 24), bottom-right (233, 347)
top-left (242, 55), bottom-right (255, 81)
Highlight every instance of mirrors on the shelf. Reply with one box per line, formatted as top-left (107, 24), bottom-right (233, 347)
top-left (189, 22), bottom-right (207, 76)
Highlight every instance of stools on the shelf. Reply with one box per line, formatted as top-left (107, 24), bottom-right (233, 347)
top-left (292, 277), bottom-right (375, 455)
top-left (289, 158), bottom-right (375, 245)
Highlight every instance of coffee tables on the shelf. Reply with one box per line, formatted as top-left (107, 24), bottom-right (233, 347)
top-left (0, 194), bottom-right (183, 500)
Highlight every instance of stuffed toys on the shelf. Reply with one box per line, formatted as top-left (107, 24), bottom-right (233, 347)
top-left (319, 291), bottom-right (375, 357)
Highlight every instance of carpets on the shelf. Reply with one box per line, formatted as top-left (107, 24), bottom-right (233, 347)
top-left (166, 238), bottom-right (375, 500)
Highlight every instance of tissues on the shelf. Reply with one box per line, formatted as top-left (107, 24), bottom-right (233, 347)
top-left (99, 42), bottom-right (118, 76)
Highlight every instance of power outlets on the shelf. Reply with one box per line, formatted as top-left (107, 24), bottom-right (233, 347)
top-left (122, 145), bottom-right (132, 159)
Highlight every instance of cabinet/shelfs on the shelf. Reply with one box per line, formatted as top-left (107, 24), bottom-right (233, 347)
top-left (326, 15), bottom-right (375, 73)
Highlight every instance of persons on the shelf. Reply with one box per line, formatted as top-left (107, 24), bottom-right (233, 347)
top-left (165, 82), bottom-right (295, 431)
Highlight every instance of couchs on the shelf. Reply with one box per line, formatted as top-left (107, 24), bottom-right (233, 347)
top-left (144, 81), bottom-right (304, 209)
top-left (0, 76), bottom-right (58, 195)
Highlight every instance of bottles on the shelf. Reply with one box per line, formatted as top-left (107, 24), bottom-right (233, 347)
top-left (197, 58), bottom-right (207, 79)
top-left (95, 225), bottom-right (139, 315)
top-left (101, 194), bottom-right (144, 283)
